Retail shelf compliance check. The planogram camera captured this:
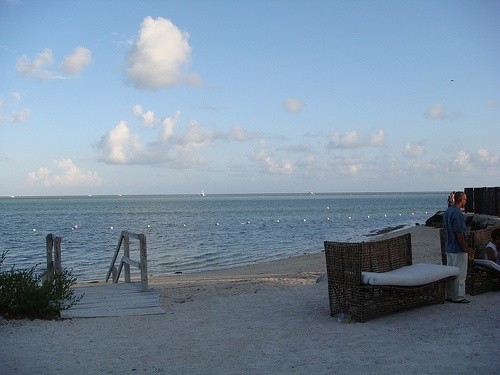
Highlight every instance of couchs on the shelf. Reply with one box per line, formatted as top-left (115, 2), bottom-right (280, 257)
top-left (323, 232), bottom-right (459, 323)
top-left (439, 225), bottom-right (500, 296)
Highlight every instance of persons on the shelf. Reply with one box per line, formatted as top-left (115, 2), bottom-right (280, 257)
top-left (451, 191), bottom-right (456, 205)
top-left (447, 193), bottom-right (452, 208)
top-left (442, 191), bottom-right (470, 303)
top-left (484, 228), bottom-right (500, 265)
